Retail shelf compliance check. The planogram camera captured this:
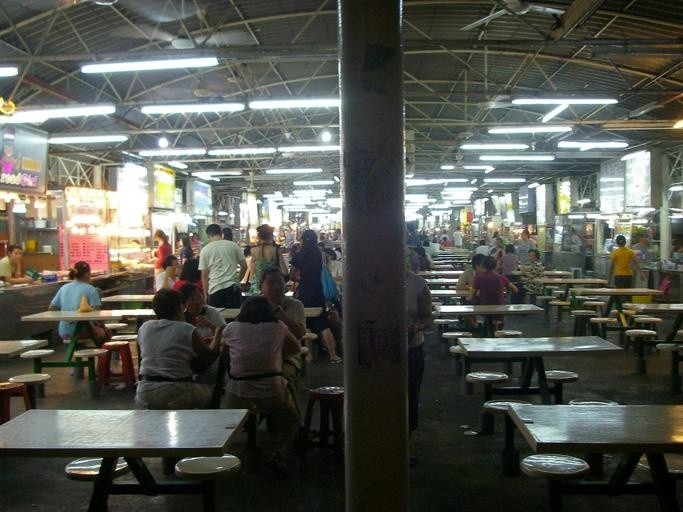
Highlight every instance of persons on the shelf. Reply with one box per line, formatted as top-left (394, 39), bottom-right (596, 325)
top-left (608, 235), bottom-right (645, 325)
top-left (0, 245), bottom-right (33, 285)
top-left (404, 223), bottom-right (547, 332)
top-left (632, 234), bottom-right (655, 289)
top-left (48, 260), bottom-right (114, 375)
top-left (564, 227), bottom-right (582, 252)
top-left (405, 271), bottom-right (434, 431)
top-left (0, 125), bottom-right (17, 176)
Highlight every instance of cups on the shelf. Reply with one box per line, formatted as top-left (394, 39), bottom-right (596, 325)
top-left (24, 240), bottom-right (36, 253)
top-left (43, 245), bottom-right (52, 253)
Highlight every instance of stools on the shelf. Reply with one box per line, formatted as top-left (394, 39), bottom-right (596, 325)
top-left (0, 241), bottom-right (341, 512)
top-left (404, 244), bottom-right (682, 512)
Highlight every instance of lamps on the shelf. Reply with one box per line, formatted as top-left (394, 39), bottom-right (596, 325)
top-left (141, 100), bottom-right (246, 116)
top-left (47, 131), bottom-right (129, 143)
top-left (459, 140), bottom-right (530, 150)
top-left (248, 96), bottom-right (341, 110)
top-left (480, 153), bottom-right (557, 162)
top-left (0, 61), bottom-right (21, 78)
top-left (541, 105), bottom-right (569, 123)
top-left (80, 57), bottom-right (220, 74)
top-left (558, 139), bottom-right (626, 150)
top-left (512, 99), bottom-right (620, 105)
top-left (136, 142), bottom-right (340, 208)
top-left (407, 165), bottom-right (594, 206)
top-left (489, 125), bottom-right (573, 134)
top-left (156, 135), bottom-right (171, 150)
top-left (319, 128), bottom-right (335, 144)
top-left (0, 100), bottom-right (117, 126)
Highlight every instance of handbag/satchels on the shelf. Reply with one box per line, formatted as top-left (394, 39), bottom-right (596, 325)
top-left (320, 251), bottom-right (337, 303)
top-left (88, 320), bottom-right (113, 347)
top-left (304, 352), bottom-right (318, 363)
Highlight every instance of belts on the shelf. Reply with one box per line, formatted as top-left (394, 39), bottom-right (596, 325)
top-left (139, 375), bottom-right (191, 382)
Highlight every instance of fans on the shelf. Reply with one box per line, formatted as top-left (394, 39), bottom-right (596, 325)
top-left (462, 0), bottom-right (566, 33)
top-left (122, 26), bottom-right (252, 51)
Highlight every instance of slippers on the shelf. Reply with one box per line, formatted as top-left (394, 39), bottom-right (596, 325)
top-left (327, 358), bottom-right (342, 363)
